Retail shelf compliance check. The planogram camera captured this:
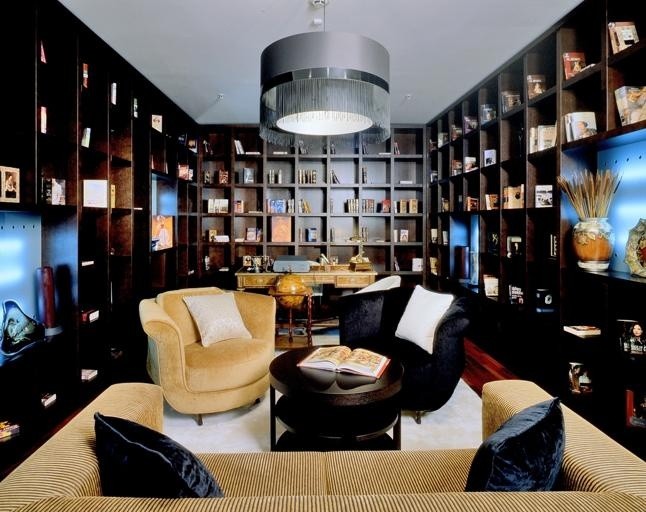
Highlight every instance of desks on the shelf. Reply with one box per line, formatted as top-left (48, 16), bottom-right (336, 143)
top-left (235, 262), bottom-right (379, 295)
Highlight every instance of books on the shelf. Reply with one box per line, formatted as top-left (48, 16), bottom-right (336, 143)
top-left (563, 319), bottom-right (646, 429)
top-left (428, 74), bottom-right (557, 313)
top-left (35, 40), bottom-right (197, 280)
top-left (80, 369), bottom-right (97, 382)
top-left (298, 345), bottom-right (392, 379)
top-left (615, 86), bottom-right (646, 126)
top-left (200, 132), bottom-right (425, 273)
top-left (0, 420), bottom-right (21, 439)
top-left (80, 309), bottom-right (100, 322)
top-left (608, 22), bottom-right (639, 54)
top-left (565, 112), bottom-right (597, 142)
top-left (563, 52), bottom-right (595, 80)
top-left (41, 391), bottom-right (57, 405)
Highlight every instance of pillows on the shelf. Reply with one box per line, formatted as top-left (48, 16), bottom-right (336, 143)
top-left (394, 284), bottom-right (457, 357)
top-left (181, 294), bottom-right (252, 349)
top-left (89, 410), bottom-right (224, 499)
top-left (464, 394), bottom-right (567, 494)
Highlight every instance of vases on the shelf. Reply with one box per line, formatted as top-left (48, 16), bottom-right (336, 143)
top-left (571, 216), bottom-right (613, 271)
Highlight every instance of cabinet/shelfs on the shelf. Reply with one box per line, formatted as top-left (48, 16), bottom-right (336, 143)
top-left (427, 1), bottom-right (646, 416)
top-left (199, 124), bottom-right (426, 275)
top-left (0, 0), bottom-right (201, 366)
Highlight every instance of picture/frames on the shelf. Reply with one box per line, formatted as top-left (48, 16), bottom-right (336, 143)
top-left (0, 166), bottom-right (21, 203)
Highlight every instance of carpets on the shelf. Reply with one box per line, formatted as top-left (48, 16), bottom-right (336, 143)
top-left (145, 329), bottom-right (500, 451)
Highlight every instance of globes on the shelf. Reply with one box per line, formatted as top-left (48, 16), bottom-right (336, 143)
top-left (268, 273), bottom-right (313, 348)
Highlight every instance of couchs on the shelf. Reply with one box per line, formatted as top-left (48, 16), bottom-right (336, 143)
top-left (0, 380), bottom-right (646, 511)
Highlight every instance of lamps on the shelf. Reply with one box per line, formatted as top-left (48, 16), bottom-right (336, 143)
top-left (254, 0), bottom-right (393, 153)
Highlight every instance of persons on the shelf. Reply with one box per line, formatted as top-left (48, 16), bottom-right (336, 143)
top-left (4, 173), bottom-right (17, 194)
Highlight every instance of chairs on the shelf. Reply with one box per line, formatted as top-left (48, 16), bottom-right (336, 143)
top-left (335, 280), bottom-right (471, 424)
top-left (137, 285), bottom-right (277, 427)
